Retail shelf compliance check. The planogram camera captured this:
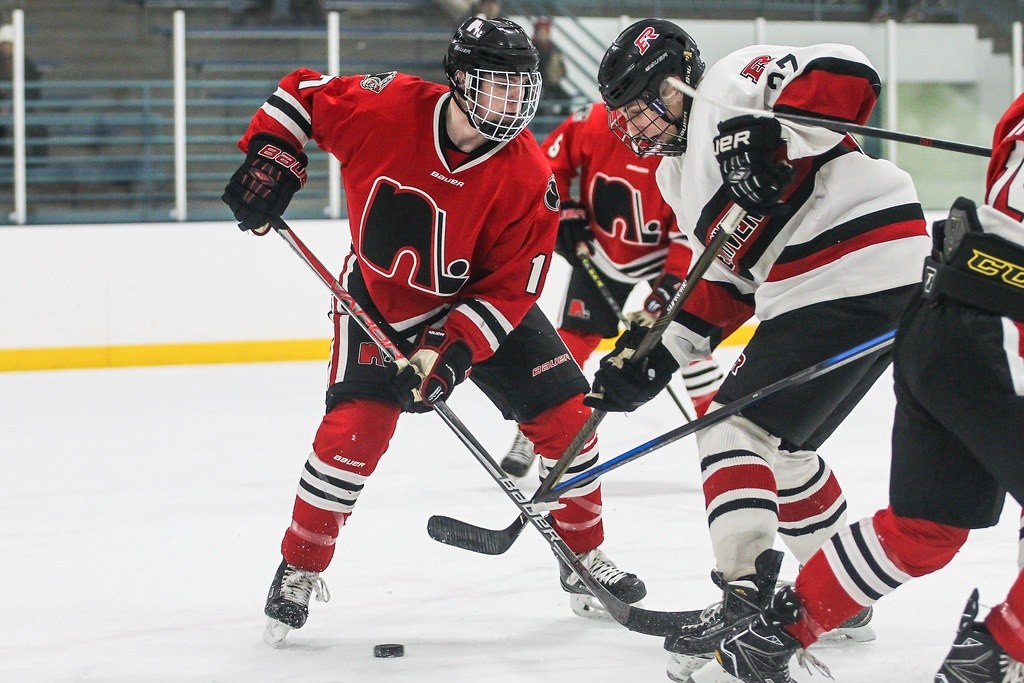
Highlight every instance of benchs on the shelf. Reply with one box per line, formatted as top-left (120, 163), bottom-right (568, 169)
top-left (0, 0), bottom-right (570, 227)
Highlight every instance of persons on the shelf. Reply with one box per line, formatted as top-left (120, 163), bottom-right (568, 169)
top-left (221, 17), bottom-right (646, 645)
top-left (0, 22), bottom-right (50, 166)
top-left (529, 16), bottom-right (572, 115)
top-left (498, 14), bottom-right (1024, 683)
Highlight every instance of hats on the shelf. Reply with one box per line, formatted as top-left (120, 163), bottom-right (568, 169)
top-left (1, 24), bottom-right (14, 44)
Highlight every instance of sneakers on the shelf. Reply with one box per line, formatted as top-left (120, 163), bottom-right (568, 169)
top-left (714, 585), bottom-right (835, 683)
top-left (816, 607), bottom-right (876, 642)
top-left (500, 427), bottom-right (539, 480)
top-left (663, 549), bottom-right (784, 683)
top-left (264, 557), bottom-right (329, 649)
top-left (551, 548), bottom-right (647, 623)
top-left (935, 588), bottom-right (1024, 683)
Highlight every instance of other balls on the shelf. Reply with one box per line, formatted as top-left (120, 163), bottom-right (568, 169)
top-left (373, 644), bottom-right (404, 658)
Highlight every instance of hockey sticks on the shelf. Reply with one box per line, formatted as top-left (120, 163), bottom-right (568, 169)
top-left (665, 76), bottom-right (992, 158)
top-left (267, 212), bottom-right (706, 639)
top-left (576, 245), bottom-right (693, 424)
top-left (530, 330), bottom-right (896, 514)
top-left (425, 204), bottom-right (752, 557)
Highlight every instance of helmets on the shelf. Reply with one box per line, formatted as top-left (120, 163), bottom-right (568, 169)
top-left (443, 15), bottom-right (543, 142)
top-left (598, 18), bottom-right (705, 159)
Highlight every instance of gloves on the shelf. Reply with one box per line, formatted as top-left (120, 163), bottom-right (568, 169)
top-left (221, 132), bottom-right (309, 232)
top-left (553, 199), bottom-right (595, 269)
top-left (627, 308), bottom-right (665, 331)
top-left (582, 318), bottom-right (681, 413)
top-left (713, 114), bottom-right (796, 217)
top-left (391, 325), bottom-right (473, 415)
